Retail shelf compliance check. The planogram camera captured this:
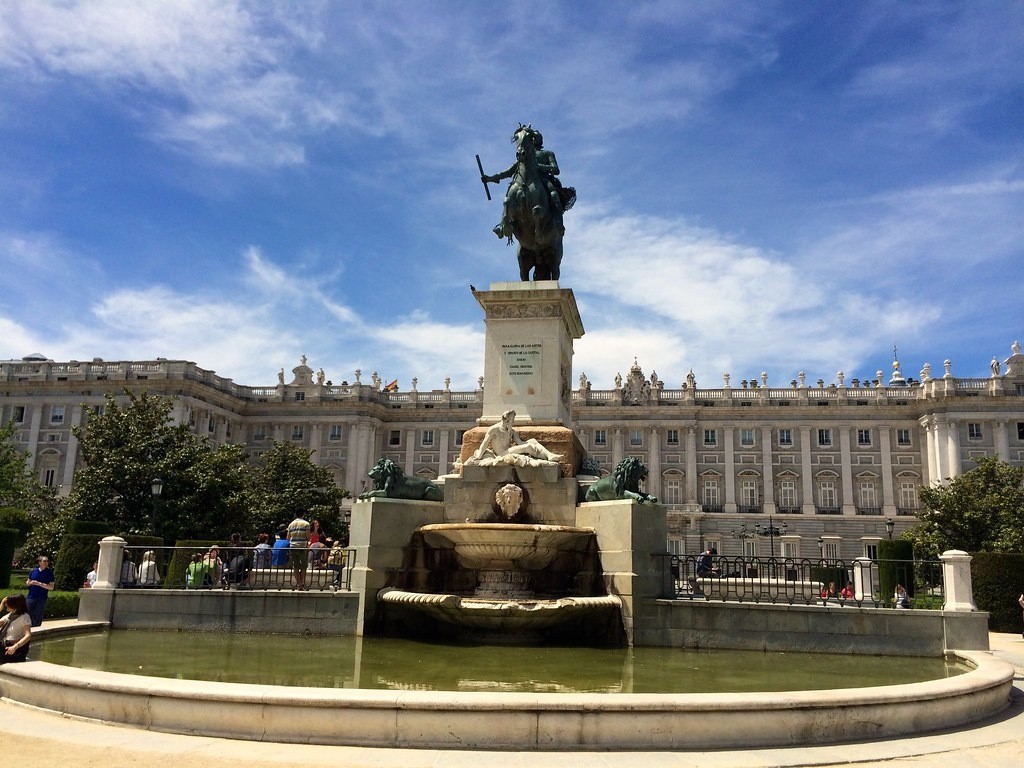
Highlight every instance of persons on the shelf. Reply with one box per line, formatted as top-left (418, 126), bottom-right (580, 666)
top-left (271, 529), bottom-right (290, 568)
top-left (309, 518), bottom-right (350, 591)
top-left (184, 532), bottom-right (249, 589)
top-left (0, 594), bottom-right (32, 665)
top-left (82, 560), bottom-right (98, 588)
top-left (1017, 594), bottom-right (1024, 640)
top-left (139, 550), bottom-right (160, 589)
top-left (476, 409), bottom-right (565, 461)
top-left (890, 584), bottom-right (909, 608)
top-left (121, 549), bottom-right (138, 588)
top-left (287, 508), bottom-right (310, 591)
top-left (696, 547), bottom-right (726, 578)
top-left (480, 130), bottom-right (566, 239)
top-left (25, 556), bottom-right (55, 627)
top-left (821, 581), bottom-right (855, 598)
top-left (253, 533), bottom-right (271, 569)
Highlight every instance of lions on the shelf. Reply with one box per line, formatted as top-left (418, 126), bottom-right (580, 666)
top-left (358, 458), bottom-right (444, 499)
top-left (585, 456), bottom-right (658, 504)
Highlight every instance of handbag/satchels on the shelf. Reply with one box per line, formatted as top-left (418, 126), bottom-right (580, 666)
top-left (901, 593), bottom-right (910, 609)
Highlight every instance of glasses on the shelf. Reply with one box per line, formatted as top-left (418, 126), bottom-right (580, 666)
top-left (40, 560), bottom-right (48, 562)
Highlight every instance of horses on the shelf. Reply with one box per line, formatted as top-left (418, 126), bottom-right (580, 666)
top-left (502, 122), bottom-right (565, 280)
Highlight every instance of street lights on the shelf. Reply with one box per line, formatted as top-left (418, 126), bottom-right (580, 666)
top-left (149, 476), bottom-right (166, 536)
top-left (886, 518), bottom-right (895, 539)
top-left (756, 514), bottom-right (788, 578)
top-left (817, 536), bottom-right (824, 566)
top-left (731, 522), bottom-right (755, 578)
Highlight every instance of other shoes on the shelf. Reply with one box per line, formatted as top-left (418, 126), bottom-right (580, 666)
top-left (298, 584), bottom-right (309, 591)
top-left (334, 580), bottom-right (341, 590)
